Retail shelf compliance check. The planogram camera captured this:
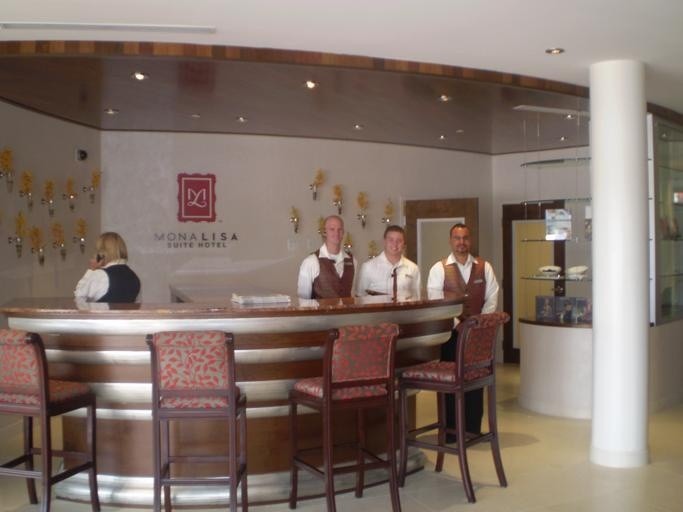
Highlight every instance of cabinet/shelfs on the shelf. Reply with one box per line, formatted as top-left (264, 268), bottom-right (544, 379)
top-left (512, 103), bottom-right (682, 422)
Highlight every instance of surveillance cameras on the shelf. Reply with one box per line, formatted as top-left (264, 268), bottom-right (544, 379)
top-left (74, 147), bottom-right (87, 162)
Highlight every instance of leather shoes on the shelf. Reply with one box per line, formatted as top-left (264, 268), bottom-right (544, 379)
top-left (444, 426), bottom-right (490, 444)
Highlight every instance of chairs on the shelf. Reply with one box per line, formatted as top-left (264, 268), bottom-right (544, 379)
top-left (0, 329), bottom-right (100, 512)
top-left (397, 312), bottom-right (511, 504)
top-left (144, 330), bottom-right (249, 512)
top-left (288, 322), bottom-right (402, 511)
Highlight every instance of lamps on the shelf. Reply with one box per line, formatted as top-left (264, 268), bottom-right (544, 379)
top-left (0, 145), bottom-right (101, 267)
top-left (288, 169), bottom-right (394, 259)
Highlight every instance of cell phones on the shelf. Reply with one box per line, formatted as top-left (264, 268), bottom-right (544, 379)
top-left (96, 254), bottom-right (104, 262)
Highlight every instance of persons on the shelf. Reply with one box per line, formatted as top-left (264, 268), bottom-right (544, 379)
top-left (558, 299), bottom-right (576, 324)
top-left (539, 298), bottom-right (553, 321)
top-left (354, 294), bottom-right (422, 305)
top-left (355, 225), bottom-right (421, 297)
top-left (72, 296), bottom-right (141, 311)
top-left (426, 222), bottom-right (500, 445)
top-left (72, 231), bottom-right (145, 304)
top-left (297, 295), bottom-right (357, 308)
top-left (578, 298), bottom-right (591, 322)
top-left (296, 214), bottom-right (359, 298)
